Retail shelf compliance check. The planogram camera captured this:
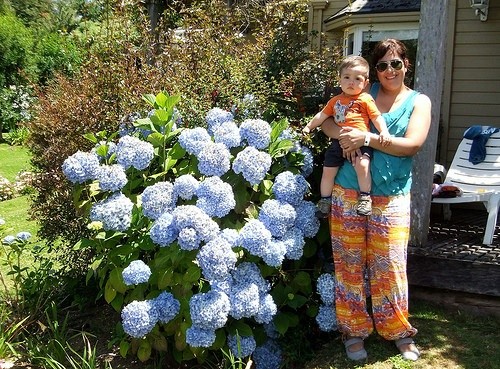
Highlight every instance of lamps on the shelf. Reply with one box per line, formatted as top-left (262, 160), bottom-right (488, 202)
top-left (470, 0), bottom-right (489, 21)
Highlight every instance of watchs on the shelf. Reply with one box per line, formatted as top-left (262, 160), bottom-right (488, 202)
top-left (364, 132), bottom-right (370, 147)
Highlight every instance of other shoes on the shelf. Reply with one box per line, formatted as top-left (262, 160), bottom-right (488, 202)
top-left (394, 338), bottom-right (421, 360)
top-left (345, 337), bottom-right (368, 361)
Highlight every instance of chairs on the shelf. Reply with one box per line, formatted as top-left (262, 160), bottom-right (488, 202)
top-left (431, 125), bottom-right (500, 244)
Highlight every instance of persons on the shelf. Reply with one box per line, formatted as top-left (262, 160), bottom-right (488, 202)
top-left (301, 55), bottom-right (392, 215)
top-left (321, 38), bottom-right (431, 361)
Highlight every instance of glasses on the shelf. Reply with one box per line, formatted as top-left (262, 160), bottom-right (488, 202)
top-left (375, 59), bottom-right (407, 73)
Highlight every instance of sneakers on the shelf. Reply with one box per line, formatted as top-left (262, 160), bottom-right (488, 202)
top-left (355, 194), bottom-right (372, 216)
top-left (317, 198), bottom-right (332, 216)
